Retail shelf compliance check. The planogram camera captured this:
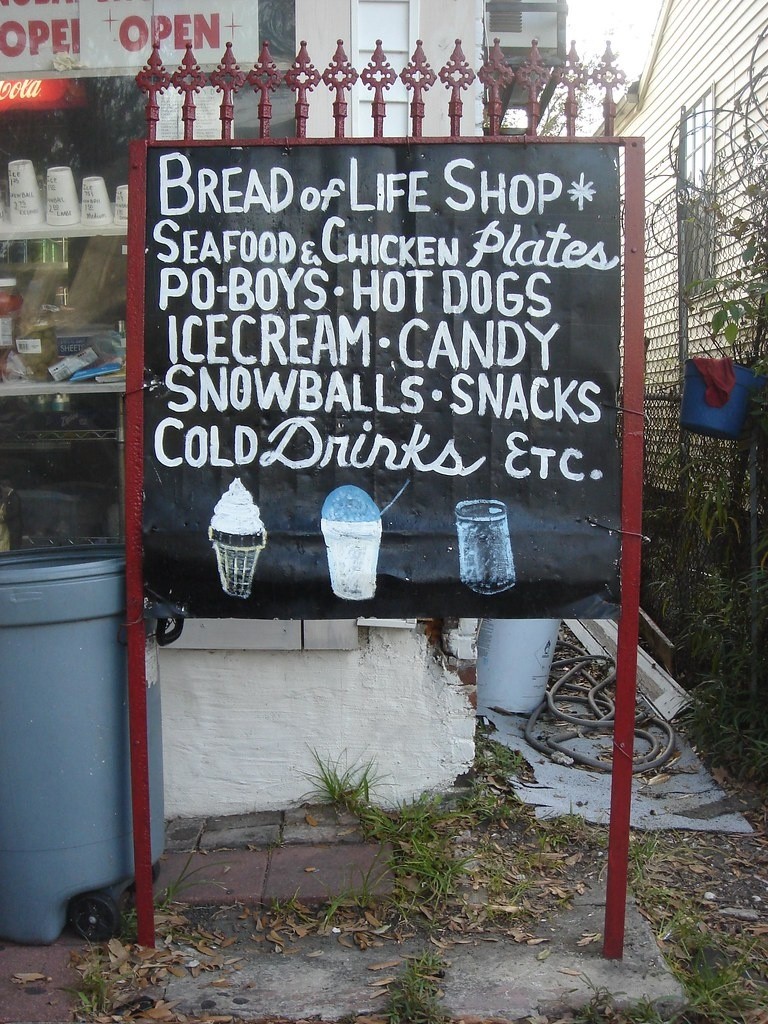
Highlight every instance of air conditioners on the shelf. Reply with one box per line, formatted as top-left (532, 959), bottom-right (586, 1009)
top-left (483, 0), bottom-right (559, 106)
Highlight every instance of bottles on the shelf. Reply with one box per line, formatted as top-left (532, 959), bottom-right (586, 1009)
top-left (0, 278), bottom-right (24, 351)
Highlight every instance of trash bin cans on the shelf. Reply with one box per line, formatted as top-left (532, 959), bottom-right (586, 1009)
top-left (0, 548), bottom-right (169, 945)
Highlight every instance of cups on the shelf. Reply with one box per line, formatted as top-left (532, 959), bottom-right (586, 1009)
top-left (81, 176), bottom-right (113, 226)
top-left (8, 160), bottom-right (45, 224)
top-left (45, 166), bottom-right (81, 225)
top-left (113, 184), bottom-right (128, 226)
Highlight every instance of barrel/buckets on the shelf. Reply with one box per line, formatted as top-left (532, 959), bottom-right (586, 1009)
top-left (0, 544), bottom-right (163, 945)
top-left (477, 619), bottom-right (562, 713)
top-left (679, 358), bottom-right (768, 440)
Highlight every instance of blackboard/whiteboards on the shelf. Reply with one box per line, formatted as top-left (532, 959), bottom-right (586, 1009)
top-left (126, 134), bottom-right (645, 619)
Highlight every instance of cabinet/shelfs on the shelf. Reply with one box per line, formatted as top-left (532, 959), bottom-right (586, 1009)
top-left (0, 175), bottom-right (128, 552)
